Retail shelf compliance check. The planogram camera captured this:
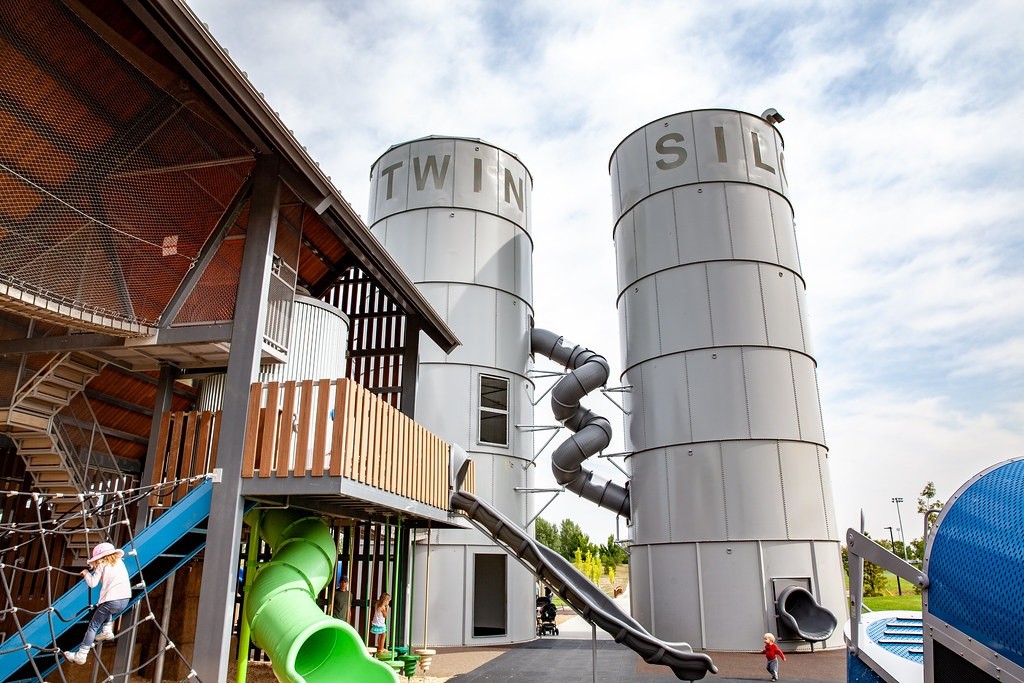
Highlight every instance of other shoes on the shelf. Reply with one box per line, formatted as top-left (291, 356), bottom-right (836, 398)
top-left (772, 677), bottom-right (777, 682)
top-left (378, 649), bottom-right (390, 655)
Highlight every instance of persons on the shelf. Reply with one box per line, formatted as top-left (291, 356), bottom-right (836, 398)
top-left (327, 576), bottom-right (353, 625)
top-left (370, 593), bottom-right (392, 654)
top-left (544, 580), bottom-right (553, 602)
top-left (760, 633), bottom-right (786, 682)
top-left (63, 542), bottom-right (132, 665)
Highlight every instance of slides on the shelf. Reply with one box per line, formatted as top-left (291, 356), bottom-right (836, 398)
top-left (452, 489), bottom-right (718, 674)
top-left (530, 328), bottom-right (837, 643)
top-left (243, 500), bottom-right (398, 683)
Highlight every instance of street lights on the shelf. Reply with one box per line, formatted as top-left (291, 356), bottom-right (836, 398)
top-left (892, 496), bottom-right (909, 563)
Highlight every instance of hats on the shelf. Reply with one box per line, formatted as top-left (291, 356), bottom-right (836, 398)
top-left (340, 576), bottom-right (348, 582)
top-left (89, 543), bottom-right (124, 563)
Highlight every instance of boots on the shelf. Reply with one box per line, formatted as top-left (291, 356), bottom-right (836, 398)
top-left (95, 622), bottom-right (114, 641)
top-left (64, 646), bottom-right (90, 665)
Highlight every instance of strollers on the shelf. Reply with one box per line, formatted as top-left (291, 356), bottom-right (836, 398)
top-left (537, 596), bottom-right (560, 636)
